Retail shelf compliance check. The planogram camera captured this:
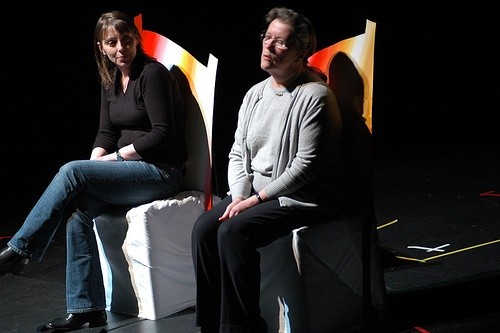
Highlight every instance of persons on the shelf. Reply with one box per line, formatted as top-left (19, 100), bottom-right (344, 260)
top-left (0, 10), bottom-right (188, 333)
top-left (191, 9), bottom-right (345, 333)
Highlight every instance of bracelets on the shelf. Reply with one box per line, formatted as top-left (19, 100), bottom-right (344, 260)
top-left (255, 193), bottom-right (263, 203)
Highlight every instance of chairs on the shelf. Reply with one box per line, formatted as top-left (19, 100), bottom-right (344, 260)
top-left (92, 12), bottom-right (221, 323)
top-left (257, 19), bottom-right (389, 333)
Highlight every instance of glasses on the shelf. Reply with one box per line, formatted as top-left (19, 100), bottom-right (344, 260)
top-left (261, 34), bottom-right (295, 48)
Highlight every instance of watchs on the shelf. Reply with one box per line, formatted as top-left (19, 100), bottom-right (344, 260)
top-left (116, 150), bottom-right (124, 161)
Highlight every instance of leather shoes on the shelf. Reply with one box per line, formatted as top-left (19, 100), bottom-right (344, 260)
top-left (0, 245), bottom-right (30, 277)
top-left (46, 309), bottom-right (108, 331)
